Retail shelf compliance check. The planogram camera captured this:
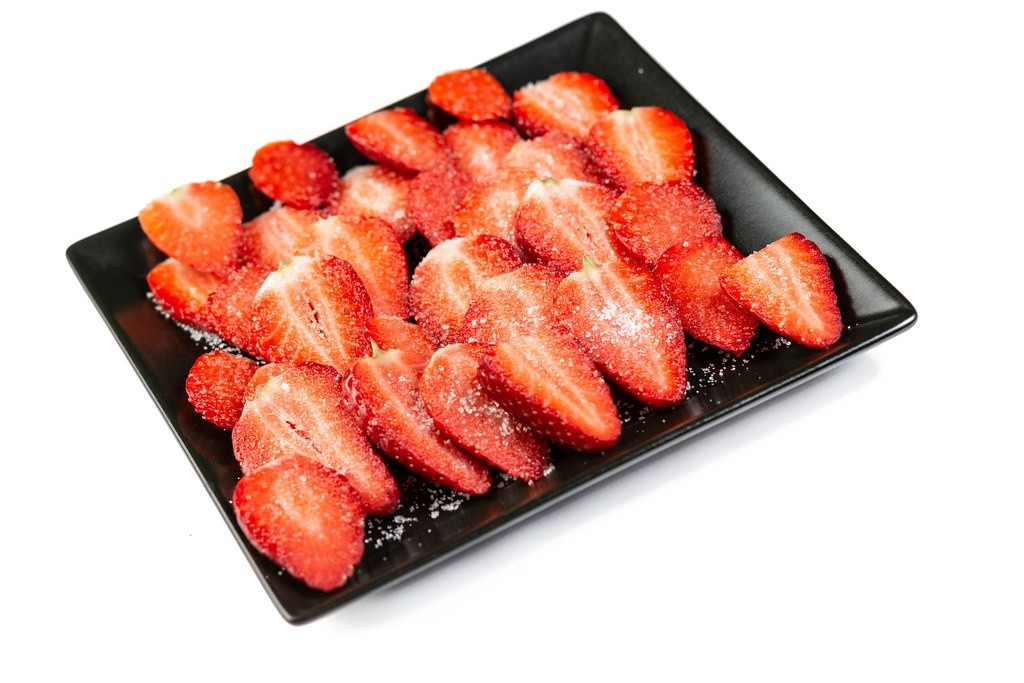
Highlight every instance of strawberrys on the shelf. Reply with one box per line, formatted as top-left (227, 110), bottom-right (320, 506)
top-left (139, 68), bottom-right (843, 589)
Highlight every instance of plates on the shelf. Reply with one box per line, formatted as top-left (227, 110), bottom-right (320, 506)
top-left (65, 10), bottom-right (920, 626)
